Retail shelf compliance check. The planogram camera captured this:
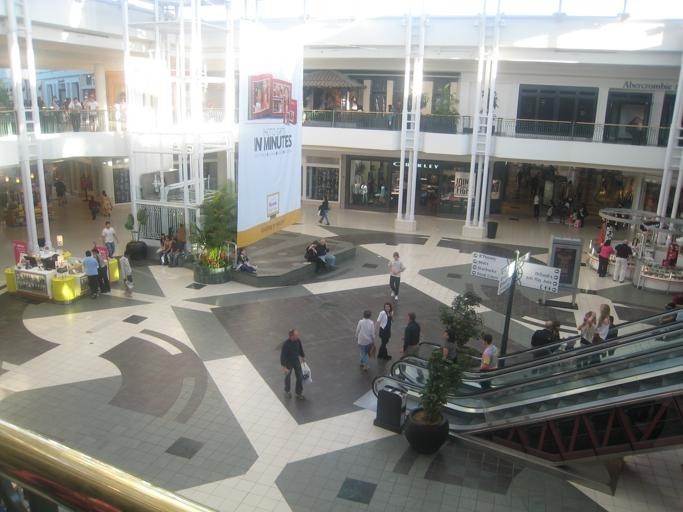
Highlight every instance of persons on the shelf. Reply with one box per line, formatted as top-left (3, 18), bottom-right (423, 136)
top-left (387, 252), bottom-right (406, 301)
top-left (119, 251), bottom-right (134, 298)
top-left (54, 181), bottom-right (65, 207)
top-left (318, 195), bottom-right (330, 225)
top-left (354, 301), bottom-right (619, 390)
top-left (89, 196), bottom-right (98, 220)
top-left (279, 328), bottom-right (306, 400)
top-left (304, 240), bottom-right (325, 266)
top-left (659, 302), bottom-right (677, 325)
top-left (237, 246), bottom-right (258, 274)
top-left (82, 250), bottom-right (99, 299)
top-left (361, 183), bottom-right (367, 204)
top-left (316, 239), bottom-right (335, 266)
top-left (92, 249), bottom-right (111, 294)
top-left (101, 220), bottom-right (119, 258)
top-left (357, 105), bottom-right (363, 112)
top-left (155, 221), bottom-right (187, 268)
top-left (515, 163), bottom-right (634, 283)
top-left (37, 94), bottom-right (126, 133)
top-left (100, 190), bottom-right (113, 218)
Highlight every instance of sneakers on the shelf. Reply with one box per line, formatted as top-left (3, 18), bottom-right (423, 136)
top-left (360, 363), bottom-right (371, 370)
top-left (283, 389), bottom-right (306, 401)
top-left (318, 219), bottom-right (330, 225)
top-left (88, 289), bottom-right (111, 299)
top-left (390, 290), bottom-right (398, 301)
top-left (377, 355), bottom-right (393, 360)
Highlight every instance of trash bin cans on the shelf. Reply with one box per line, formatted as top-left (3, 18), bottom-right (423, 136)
top-left (487, 222), bottom-right (498, 239)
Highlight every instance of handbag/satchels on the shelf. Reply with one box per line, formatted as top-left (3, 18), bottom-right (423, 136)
top-left (124, 279), bottom-right (136, 290)
top-left (368, 343), bottom-right (377, 359)
top-left (300, 358), bottom-right (313, 386)
top-left (317, 209), bottom-right (322, 216)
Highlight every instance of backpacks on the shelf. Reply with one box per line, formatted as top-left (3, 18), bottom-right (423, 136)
top-left (530, 328), bottom-right (546, 351)
